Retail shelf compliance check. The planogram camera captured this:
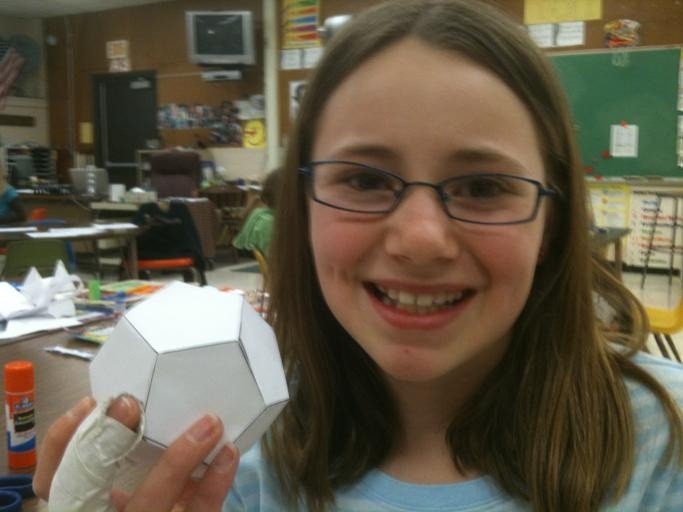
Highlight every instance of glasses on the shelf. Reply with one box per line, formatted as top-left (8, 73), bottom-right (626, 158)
top-left (298, 160), bottom-right (559, 225)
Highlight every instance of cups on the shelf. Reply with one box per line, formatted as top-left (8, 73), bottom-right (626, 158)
top-left (106, 184), bottom-right (126, 202)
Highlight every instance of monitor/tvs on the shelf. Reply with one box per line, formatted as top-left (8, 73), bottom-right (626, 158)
top-left (184, 11), bottom-right (256, 66)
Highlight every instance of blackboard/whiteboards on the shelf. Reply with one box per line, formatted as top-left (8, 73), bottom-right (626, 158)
top-left (546, 44), bottom-right (683, 179)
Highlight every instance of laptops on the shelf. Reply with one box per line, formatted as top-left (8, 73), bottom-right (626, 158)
top-left (68, 168), bottom-right (113, 197)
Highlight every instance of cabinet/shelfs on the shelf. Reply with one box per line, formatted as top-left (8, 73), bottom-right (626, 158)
top-left (134, 149), bottom-right (243, 264)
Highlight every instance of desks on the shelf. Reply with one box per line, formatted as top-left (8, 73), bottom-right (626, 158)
top-left (633, 186), bottom-right (683, 289)
top-left (90, 198), bottom-right (217, 270)
top-left (0, 319), bottom-right (168, 512)
top-left (595, 227), bottom-right (631, 281)
top-left (0, 220), bottom-right (151, 280)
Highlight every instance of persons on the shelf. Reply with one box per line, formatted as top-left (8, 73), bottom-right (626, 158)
top-left (231, 164), bottom-right (286, 267)
top-left (27, 0), bottom-right (682, 512)
top-left (0, 175), bottom-right (26, 224)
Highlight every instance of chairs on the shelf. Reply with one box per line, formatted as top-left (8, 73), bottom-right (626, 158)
top-left (23, 219), bottom-right (77, 274)
top-left (645, 292), bottom-right (683, 361)
top-left (118, 199), bottom-right (207, 287)
top-left (0, 207), bottom-right (48, 254)
top-left (253, 248), bottom-right (273, 320)
top-left (0, 239), bottom-right (69, 274)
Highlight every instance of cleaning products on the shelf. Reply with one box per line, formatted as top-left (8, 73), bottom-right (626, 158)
top-left (5, 362), bottom-right (39, 470)
